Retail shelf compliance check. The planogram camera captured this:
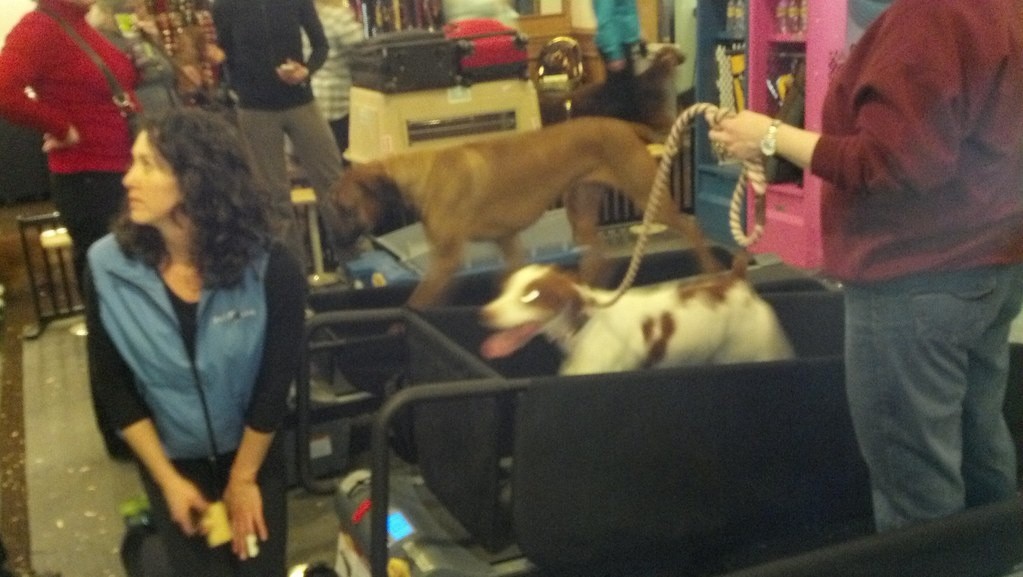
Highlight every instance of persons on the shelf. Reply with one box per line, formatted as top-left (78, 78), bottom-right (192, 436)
top-left (705, 0), bottom-right (1023, 531)
top-left (211, 0), bottom-right (373, 275)
top-left (81, 105), bottom-right (307, 577)
top-left (0, 0), bottom-right (146, 460)
top-left (593, 0), bottom-right (642, 120)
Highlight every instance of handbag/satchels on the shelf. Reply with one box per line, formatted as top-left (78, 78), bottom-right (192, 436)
top-left (117, 80), bottom-right (183, 136)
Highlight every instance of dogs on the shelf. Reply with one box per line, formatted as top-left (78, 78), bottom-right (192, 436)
top-left (318, 114), bottom-right (726, 333)
top-left (476, 244), bottom-right (797, 376)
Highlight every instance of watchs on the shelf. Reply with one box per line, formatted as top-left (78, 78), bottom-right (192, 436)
top-left (760, 117), bottom-right (782, 157)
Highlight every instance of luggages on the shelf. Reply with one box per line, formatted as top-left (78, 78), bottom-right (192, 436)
top-left (439, 20), bottom-right (533, 84)
top-left (345, 30), bottom-right (469, 94)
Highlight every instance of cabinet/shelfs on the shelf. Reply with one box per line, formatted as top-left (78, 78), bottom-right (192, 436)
top-left (694, 0), bottom-right (847, 274)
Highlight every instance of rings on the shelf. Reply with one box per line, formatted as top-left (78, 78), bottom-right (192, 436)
top-left (711, 142), bottom-right (725, 155)
